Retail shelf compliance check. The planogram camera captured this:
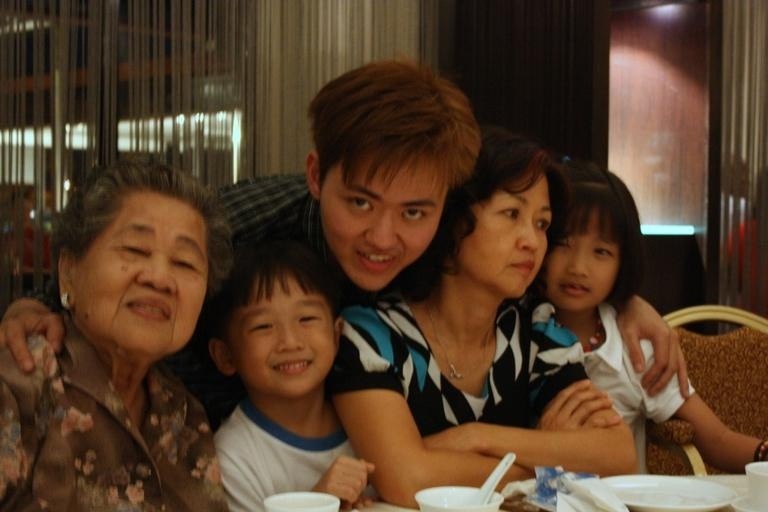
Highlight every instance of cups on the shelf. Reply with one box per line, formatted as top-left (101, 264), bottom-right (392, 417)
top-left (264, 489), bottom-right (341, 512)
top-left (745, 461), bottom-right (768, 512)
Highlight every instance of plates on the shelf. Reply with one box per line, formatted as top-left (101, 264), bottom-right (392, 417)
top-left (598, 473), bottom-right (739, 512)
top-left (730, 495), bottom-right (752, 512)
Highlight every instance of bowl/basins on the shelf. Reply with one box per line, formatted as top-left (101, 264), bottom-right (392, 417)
top-left (414, 485), bottom-right (504, 512)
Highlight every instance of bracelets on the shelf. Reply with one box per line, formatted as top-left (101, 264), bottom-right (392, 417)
top-left (755, 440), bottom-right (767, 463)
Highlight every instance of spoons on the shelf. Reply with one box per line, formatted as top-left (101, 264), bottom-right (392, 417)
top-left (462, 451), bottom-right (517, 509)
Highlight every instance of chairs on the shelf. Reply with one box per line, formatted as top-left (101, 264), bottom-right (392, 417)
top-left (645, 304), bottom-right (768, 477)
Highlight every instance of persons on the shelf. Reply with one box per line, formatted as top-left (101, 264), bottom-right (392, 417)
top-left (538, 163), bottom-right (768, 479)
top-left (724, 165), bottom-right (767, 326)
top-left (206, 247), bottom-right (380, 512)
top-left (0, 58), bottom-right (689, 435)
top-left (0, 155), bottom-right (235, 512)
top-left (325, 132), bottom-right (638, 507)
top-left (2, 194), bottom-right (53, 270)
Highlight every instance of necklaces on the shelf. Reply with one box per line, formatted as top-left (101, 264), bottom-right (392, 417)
top-left (555, 315), bottom-right (605, 352)
top-left (420, 298), bottom-right (499, 379)
